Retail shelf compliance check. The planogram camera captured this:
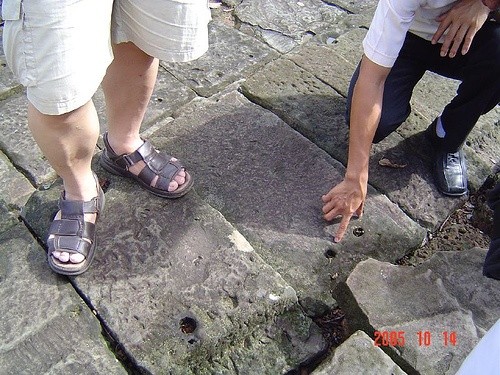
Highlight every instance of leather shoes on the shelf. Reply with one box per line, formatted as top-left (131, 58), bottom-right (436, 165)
top-left (428, 116), bottom-right (468, 198)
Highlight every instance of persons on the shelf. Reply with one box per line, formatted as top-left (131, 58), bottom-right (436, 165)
top-left (321, 0), bottom-right (500, 243)
top-left (1, 0), bottom-right (212, 276)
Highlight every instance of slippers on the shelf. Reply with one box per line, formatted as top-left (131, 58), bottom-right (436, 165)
top-left (101, 132), bottom-right (195, 199)
top-left (46, 173), bottom-right (108, 276)
